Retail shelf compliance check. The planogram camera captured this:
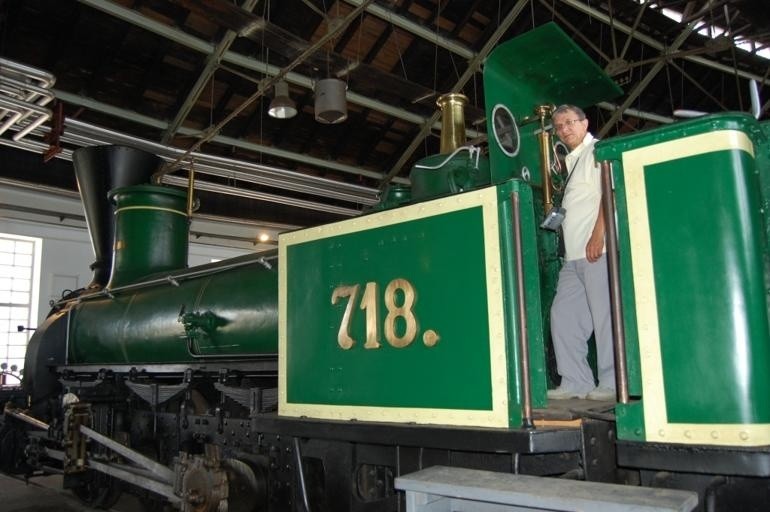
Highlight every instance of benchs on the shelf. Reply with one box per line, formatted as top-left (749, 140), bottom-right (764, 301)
top-left (393, 460), bottom-right (698, 512)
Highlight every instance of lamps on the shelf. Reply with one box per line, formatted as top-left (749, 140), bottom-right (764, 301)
top-left (269, 80), bottom-right (299, 117)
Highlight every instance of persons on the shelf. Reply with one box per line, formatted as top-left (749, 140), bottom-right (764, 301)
top-left (545, 105), bottom-right (619, 402)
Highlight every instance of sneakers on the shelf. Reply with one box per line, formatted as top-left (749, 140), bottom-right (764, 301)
top-left (586, 383), bottom-right (616, 402)
top-left (544, 384), bottom-right (581, 400)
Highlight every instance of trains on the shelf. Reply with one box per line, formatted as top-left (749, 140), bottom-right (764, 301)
top-left (0, 21), bottom-right (770, 512)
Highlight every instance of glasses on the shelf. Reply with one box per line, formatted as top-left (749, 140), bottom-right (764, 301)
top-left (554, 119), bottom-right (583, 131)
